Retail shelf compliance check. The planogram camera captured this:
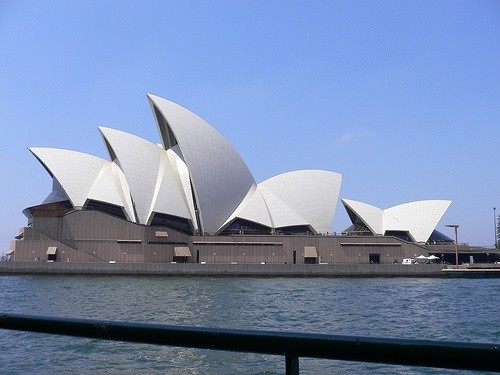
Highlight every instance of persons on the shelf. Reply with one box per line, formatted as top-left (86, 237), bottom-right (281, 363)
top-left (231, 228), bottom-right (358, 236)
top-left (0, 253), bottom-right (71, 263)
top-left (370, 256), bottom-right (499, 265)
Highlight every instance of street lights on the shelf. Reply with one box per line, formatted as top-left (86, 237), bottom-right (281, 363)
top-left (493, 207), bottom-right (496, 246)
top-left (445, 224), bottom-right (459, 265)
